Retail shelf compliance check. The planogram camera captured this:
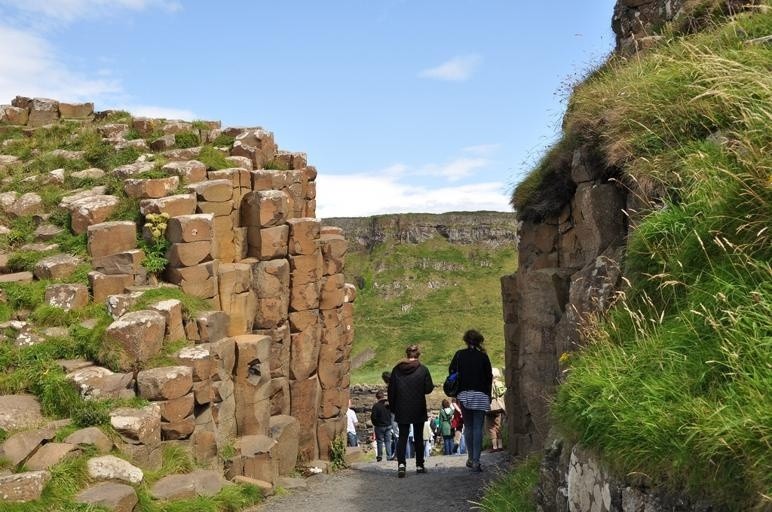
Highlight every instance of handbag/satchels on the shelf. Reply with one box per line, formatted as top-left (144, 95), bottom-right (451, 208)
top-left (443, 372), bottom-right (461, 397)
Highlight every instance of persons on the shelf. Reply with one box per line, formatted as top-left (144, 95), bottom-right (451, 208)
top-left (370, 391), bottom-right (395, 462)
top-left (381, 371), bottom-right (394, 412)
top-left (386, 342), bottom-right (434, 478)
top-left (390, 398), bottom-right (466, 458)
top-left (490, 367), bottom-right (508, 453)
top-left (346, 400), bottom-right (360, 448)
top-left (448, 329), bottom-right (493, 472)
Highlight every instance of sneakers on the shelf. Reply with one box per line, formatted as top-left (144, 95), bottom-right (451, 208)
top-left (487, 446), bottom-right (504, 453)
top-left (377, 457), bottom-right (427, 477)
top-left (466, 460), bottom-right (481, 473)
